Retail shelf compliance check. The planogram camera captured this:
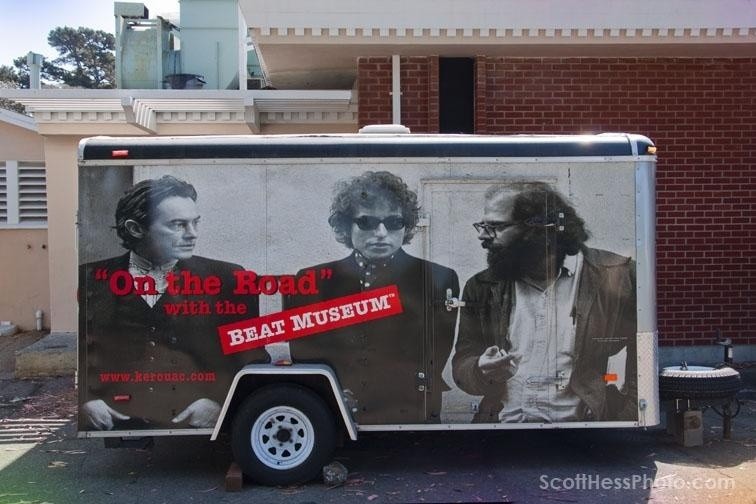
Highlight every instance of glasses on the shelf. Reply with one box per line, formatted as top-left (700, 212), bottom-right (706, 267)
top-left (472, 220), bottom-right (519, 239)
top-left (350, 215), bottom-right (407, 232)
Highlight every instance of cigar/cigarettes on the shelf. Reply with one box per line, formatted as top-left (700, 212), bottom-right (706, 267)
top-left (501, 348), bottom-right (517, 369)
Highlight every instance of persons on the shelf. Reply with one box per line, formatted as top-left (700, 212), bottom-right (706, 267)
top-left (284, 167), bottom-right (462, 423)
top-left (448, 180), bottom-right (638, 424)
top-left (77, 173), bottom-right (269, 432)
